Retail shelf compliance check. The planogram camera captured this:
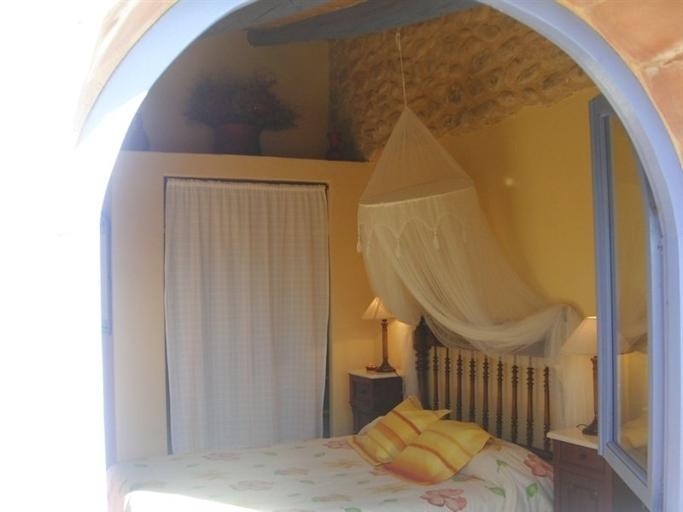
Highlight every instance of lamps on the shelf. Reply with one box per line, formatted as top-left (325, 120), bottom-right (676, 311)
top-left (562, 315), bottom-right (629, 438)
top-left (360, 297), bottom-right (401, 373)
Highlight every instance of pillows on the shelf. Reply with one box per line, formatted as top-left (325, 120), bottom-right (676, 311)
top-left (350, 392), bottom-right (492, 482)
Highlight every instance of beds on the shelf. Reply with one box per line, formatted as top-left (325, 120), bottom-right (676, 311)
top-left (117, 418), bottom-right (555, 510)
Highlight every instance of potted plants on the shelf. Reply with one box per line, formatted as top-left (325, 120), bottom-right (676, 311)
top-left (179, 67), bottom-right (300, 157)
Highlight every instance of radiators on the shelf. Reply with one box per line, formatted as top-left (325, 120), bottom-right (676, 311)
top-left (427, 346), bottom-right (557, 452)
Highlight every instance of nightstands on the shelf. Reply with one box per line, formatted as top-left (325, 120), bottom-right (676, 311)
top-left (544, 433), bottom-right (645, 510)
top-left (348, 372), bottom-right (401, 432)
